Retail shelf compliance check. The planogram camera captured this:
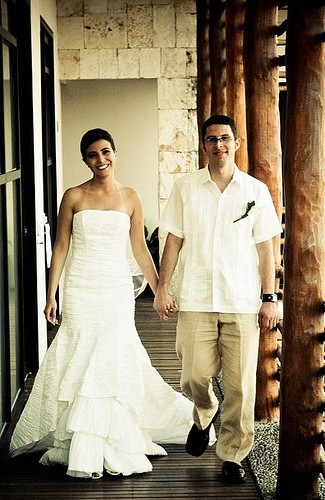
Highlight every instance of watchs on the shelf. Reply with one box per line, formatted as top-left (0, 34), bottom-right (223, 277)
top-left (263, 293), bottom-right (278, 303)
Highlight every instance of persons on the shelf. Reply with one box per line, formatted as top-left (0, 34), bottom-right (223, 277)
top-left (42, 127), bottom-right (180, 479)
top-left (153, 114), bottom-right (281, 485)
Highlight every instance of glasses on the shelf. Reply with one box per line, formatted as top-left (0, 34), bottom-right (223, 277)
top-left (204, 136), bottom-right (234, 142)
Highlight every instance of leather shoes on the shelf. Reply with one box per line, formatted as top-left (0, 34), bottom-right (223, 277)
top-left (220, 461), bottom-right (248, 484)
top-left (185, 405), bottom-right (221, 458)
